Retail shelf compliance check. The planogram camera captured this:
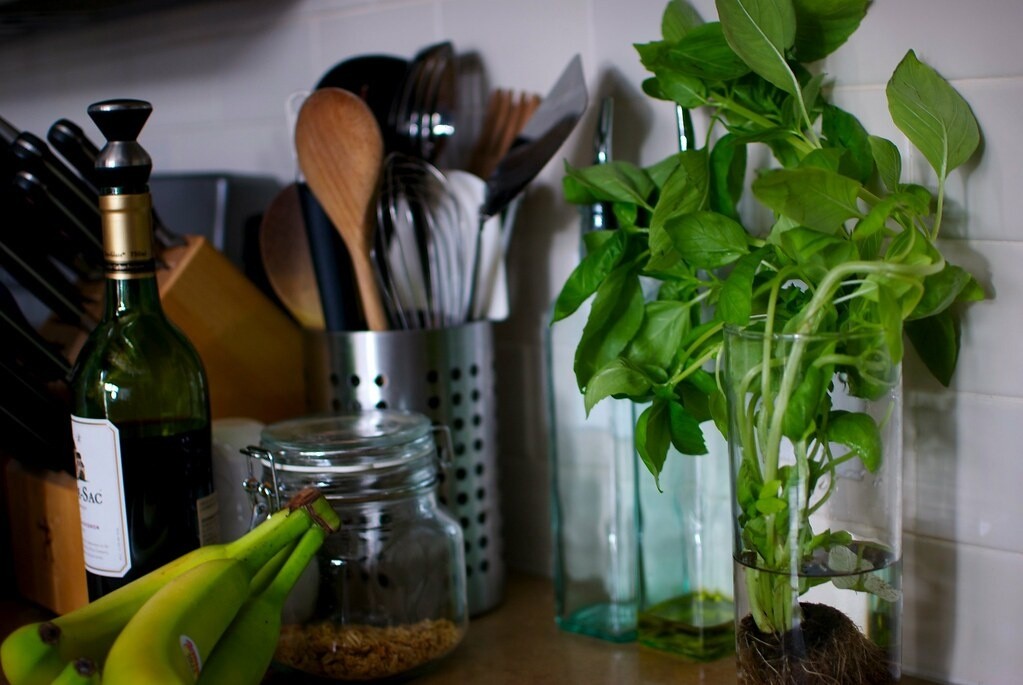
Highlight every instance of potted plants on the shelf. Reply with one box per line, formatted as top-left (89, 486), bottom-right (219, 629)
top-left (546, 2), bottom-right (992, 685)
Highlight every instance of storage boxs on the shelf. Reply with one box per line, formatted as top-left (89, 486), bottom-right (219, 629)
top-left (0, 235), bottom-right (309, 618)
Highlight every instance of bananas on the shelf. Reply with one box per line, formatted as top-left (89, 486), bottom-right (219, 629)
top-left (0, 482), bottom-right (339, 685)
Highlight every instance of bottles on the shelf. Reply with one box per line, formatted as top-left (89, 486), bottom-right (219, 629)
top-left (254, 409), bottom-right (469, 681)
top-left (625, 104), bottom-right (744, 660)
top-left (68, 169), bottom-right (217, 612)
top-left (543, 89), bottom-right (654, 646)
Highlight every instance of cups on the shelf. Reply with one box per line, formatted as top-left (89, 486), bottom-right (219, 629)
top-left (725, 311), bottom-right (911, 685)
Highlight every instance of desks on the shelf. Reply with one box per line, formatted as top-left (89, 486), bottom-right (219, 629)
top-left (3, 573), bottom-right (915, 685)
